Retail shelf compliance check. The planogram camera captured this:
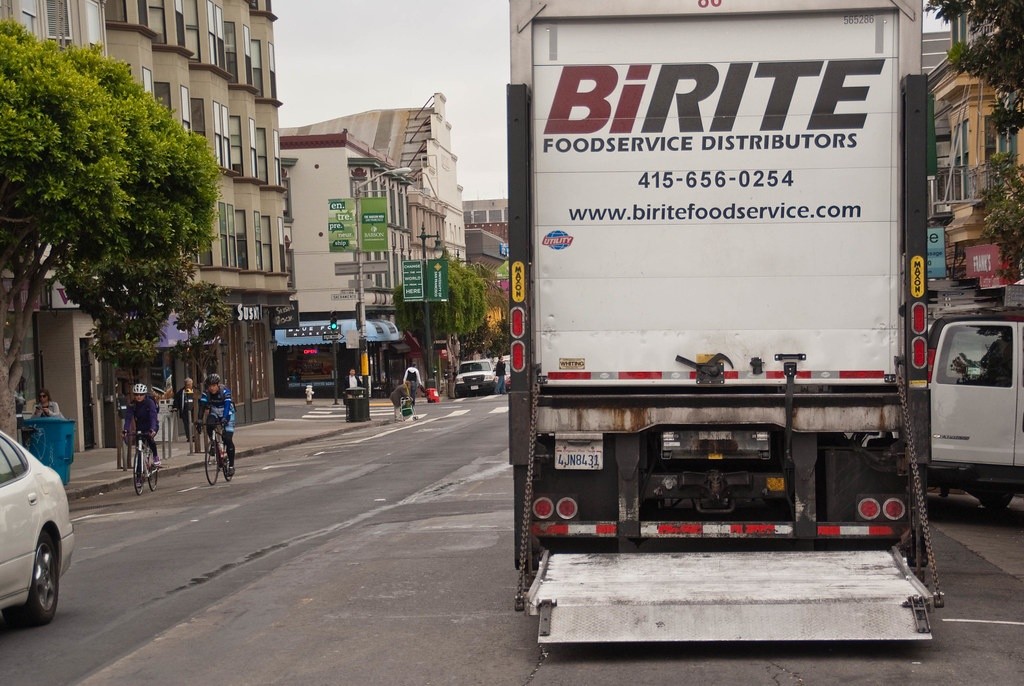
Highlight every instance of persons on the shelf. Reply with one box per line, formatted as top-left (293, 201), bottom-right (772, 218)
top-left (343, 369), bottom-right (363, 391)
top-left (390, 381), bottom-right (412, 422)
top-left (31, 389), bottom-right (64, 418)
top-left (403, 363), bottom-right (421, 406)
top-left (495, 355), bottom-right (507, 394)
top-left (196, 373), bottom-right (237, 476)
top-left (173, 377), bottom-right (208, 442)
top-left (14, 376), bottom-right (26, 428)
top-left (123, 383), bottom-right (161, 487)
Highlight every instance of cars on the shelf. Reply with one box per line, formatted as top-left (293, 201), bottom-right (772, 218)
top-left (0, 432), bottom-right (73, 627)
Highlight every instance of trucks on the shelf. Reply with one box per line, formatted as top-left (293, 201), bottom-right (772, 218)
top-left (509, 0), bottom-right (947, 646)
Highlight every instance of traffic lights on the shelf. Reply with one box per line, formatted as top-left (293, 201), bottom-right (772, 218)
top-left (329, 310), bottom-right (339, 333)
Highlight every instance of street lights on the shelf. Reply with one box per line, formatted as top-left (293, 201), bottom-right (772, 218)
top-left (352, 166), bottom-right (417, 405)
top-left (415, 221), bottom-right (455, 409)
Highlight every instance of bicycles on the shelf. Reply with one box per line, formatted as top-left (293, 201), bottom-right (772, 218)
top-left (123, 430), bottom-right (168, 496)
top-left (194, 421), bottom-right (235, 486)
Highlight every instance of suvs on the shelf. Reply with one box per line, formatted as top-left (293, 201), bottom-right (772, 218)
top-left (495, 356), bottom-right (510, 388)
top-left (455, 360), bottom-right (499, 398)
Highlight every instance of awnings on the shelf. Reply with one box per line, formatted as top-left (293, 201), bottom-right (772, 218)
top-left (274, 318), bottom-right (400, 346)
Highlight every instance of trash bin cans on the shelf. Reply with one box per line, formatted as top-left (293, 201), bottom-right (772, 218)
top-left (345, 387), bottom-right (367, 422)
top-left (23, 418), bottom-right (77, 485)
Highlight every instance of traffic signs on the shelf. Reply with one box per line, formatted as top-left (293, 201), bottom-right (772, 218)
top-left (322, 334), bottom-right (345, 343)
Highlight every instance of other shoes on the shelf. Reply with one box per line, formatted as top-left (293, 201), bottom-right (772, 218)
top-left (210, 446), bottom-right (218, 457)
top-left (153, 456), bottom-right (162, 465)
top-left (227, 468), bottom-right (236, 475)
top-left (136, 478), bottom-right (143, 488)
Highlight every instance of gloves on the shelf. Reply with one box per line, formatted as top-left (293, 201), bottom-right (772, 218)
top-left (221, 418), bottom-right (227, 429)
top-left (195, 420), bottom-right (204, 434)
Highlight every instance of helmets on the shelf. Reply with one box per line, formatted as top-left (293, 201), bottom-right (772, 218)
top-left (132, 384), bottom-right (147, 395)
top-left (205, 373), bottom-right (220, 385)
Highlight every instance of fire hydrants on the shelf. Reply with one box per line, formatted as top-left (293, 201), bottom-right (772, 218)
top-left (305, 385), bottom-right (320, 407)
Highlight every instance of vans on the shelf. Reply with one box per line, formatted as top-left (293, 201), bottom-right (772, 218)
top-left (922, 304), bottom-right (1024, 511)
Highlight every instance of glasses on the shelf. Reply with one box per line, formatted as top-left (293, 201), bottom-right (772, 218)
top-left (39, 394), bottom-right (46, 399)
